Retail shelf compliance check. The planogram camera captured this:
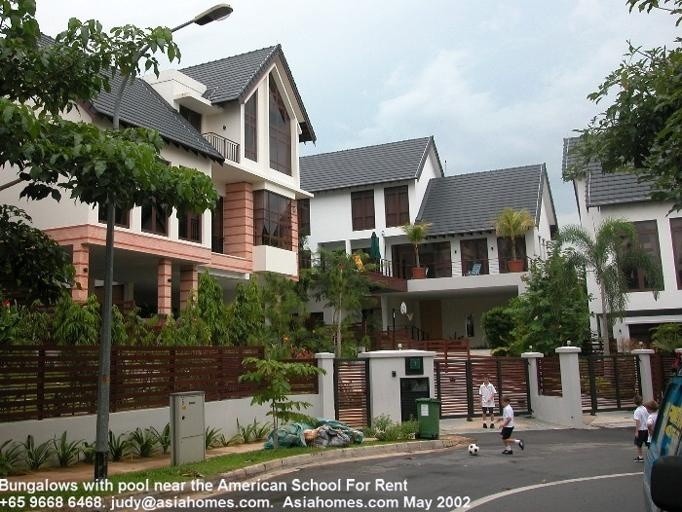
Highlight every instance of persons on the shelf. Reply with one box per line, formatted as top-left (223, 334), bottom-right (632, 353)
top-left (630, 395), bottom-right (649, 463)
top-left (494, 394), bottom-right (525, 456)
top-left (479, 375), bottom-right (497, 428)
top-left (642, 398), bottom-right (659, 449)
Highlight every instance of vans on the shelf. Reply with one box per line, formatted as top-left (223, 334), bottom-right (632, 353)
top-left (643, 345), bottom-right (682, 512)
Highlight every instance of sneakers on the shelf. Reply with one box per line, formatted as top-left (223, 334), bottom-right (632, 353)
top-left (482, 422), bottom-right (495, 428)
top-left (501, 448), bottom-right (514, 455)
top-left (633, 456), bottom-right (644, 463)
top-left (518, 439), bottom-right (524, 450)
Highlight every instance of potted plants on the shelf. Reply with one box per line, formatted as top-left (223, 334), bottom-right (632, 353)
top-left (402, 221), bottom-right (431, 279)
top-left (489, 207), bottom-right (538, 272)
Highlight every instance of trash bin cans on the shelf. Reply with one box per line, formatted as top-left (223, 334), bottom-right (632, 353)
top-left (416, 397), bottom-right (442, 439)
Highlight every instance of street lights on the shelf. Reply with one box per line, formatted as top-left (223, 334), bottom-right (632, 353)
top-left (390, 307), bottom-right (398, 344)
top-left (93, 1), bottom-right (235, 481)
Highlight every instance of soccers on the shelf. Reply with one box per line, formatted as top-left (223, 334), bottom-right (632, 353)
top-left (468, 443), bottom-right (480, 455)
